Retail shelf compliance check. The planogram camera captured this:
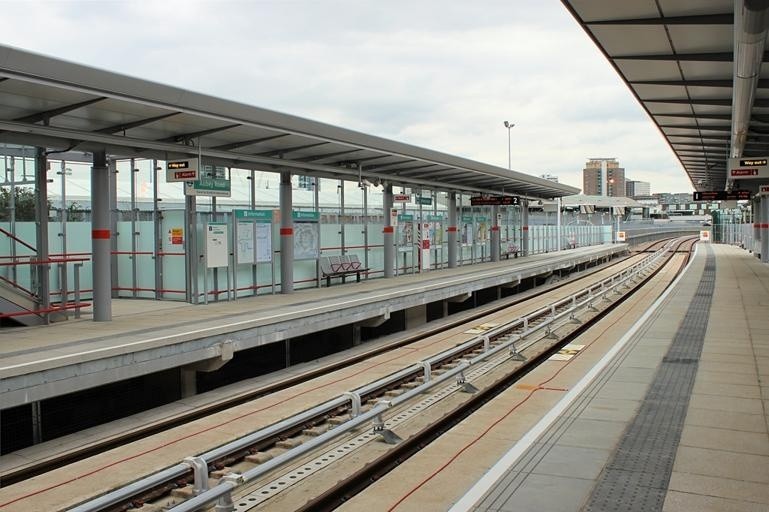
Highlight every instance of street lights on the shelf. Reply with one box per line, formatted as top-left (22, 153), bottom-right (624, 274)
top-left (504, 121), bottom-right (515, 170)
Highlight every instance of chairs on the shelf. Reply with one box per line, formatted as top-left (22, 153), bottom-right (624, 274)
top-left (320, 254), bottom-right (369, 286)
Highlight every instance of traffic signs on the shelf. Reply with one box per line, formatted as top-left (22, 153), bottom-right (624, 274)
top-left (415, 196), bottom-right (432, 206)
top-left (184, 177), bottom-right (231, 198)
